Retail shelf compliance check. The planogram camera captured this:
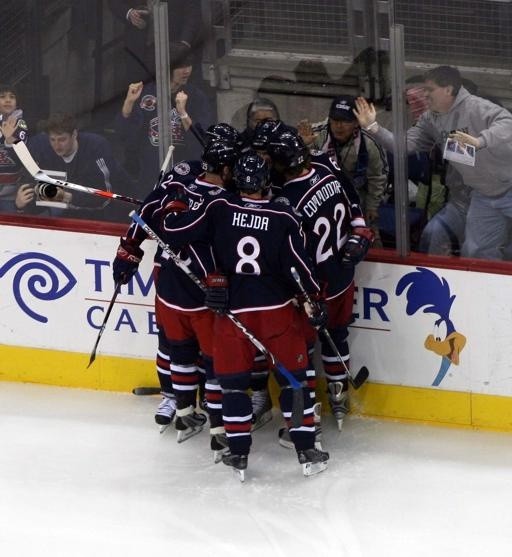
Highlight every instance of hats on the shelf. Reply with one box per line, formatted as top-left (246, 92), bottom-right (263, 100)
top-left (329, 94), bottom-right (359, 121)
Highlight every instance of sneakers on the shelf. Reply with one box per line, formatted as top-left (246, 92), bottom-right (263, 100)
top-left (211, 433), bottom-right (229, 450)
top-left (329, 396), bottom-right (350, 419)
top-left (222, 454), bottom-right (247, 469)
top-left (296, 447), bottom-right (329, 464)
top-left (199, 390), bottom-right (210, 413)
top-left (155, 391), bottom-right (177, 425)
top-left (250, 389), bottom-right (272, 424)
top-left (176, 410), bottom-right (207, 430)
top-left (279, 425), bottom-right (322, 442)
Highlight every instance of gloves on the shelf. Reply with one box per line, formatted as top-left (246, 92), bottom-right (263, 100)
top-left (113, 235), bottom-right (144, 293)
top-left (338, 226), bottom-right (376, 270)
top-left (204, 273), bottom-right (234, 314)
top-left (308, 297), bottom-right (329, 337)
top-left (160, 184), bottom-right (189, 217)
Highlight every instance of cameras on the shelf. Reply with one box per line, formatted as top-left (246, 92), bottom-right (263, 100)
top-left (24, 180), bottom-right (58, 201)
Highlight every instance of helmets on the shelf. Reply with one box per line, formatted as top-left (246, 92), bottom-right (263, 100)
top-left (250, 119), bottom-right (287, 150)
top-left (231, 152), bottom-right (273, 196)
top-left (201, 141), bottom-right (241, 174)
top-left (265, 128), bottom-right (309, 167)
top-left (203, 123), bottom-right (241, 153)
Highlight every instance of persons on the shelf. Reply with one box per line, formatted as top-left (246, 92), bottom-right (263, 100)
top-left (107, 1), bottom-right (190, 83)
top-left (108, 92), bottom-right (392, 474)
top-left (120, 37), bottom-right (214, 196)
top-left (350, 62), bottom-right (512, 262)
top-left (0, 83), bottom-right (31, 215)
top-left (14, 108), bottom-right (131, 228)
